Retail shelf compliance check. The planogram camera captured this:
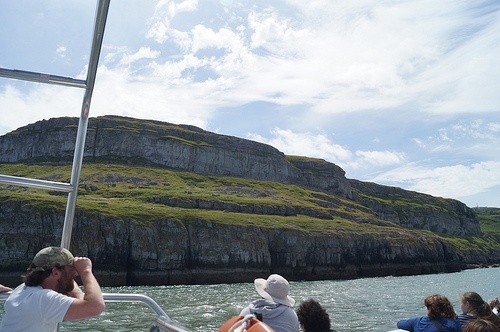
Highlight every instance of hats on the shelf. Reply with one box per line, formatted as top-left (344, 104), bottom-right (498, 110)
top-left (255, 275), bottom-right (295, 307)
top-left (30, 247), bottom-right (75, 271)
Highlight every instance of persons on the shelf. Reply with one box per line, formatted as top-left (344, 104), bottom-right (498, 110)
top-left (0, 246), bottom-right (105, 332)
top-left (397, 295), bottom-right (461, 332)
top-left (240, 274), bottom-right (299, 332)
top-left (458, 292), bottom-right (500, 332)
top-left (296, 299), bottom-right (336, 332)
